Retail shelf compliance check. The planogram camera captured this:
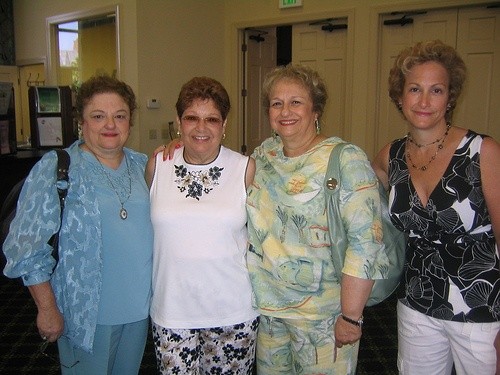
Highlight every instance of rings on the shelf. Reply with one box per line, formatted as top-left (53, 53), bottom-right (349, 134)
top-left (43, 337), bottom-right (48, 339)
top-left (164, 145), bottom-right (167, 148)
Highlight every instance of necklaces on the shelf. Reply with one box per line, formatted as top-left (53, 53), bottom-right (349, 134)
top-left (406, 122), bottom-right (451, 170)
top-left (93, 152), bottom-right (131, 220)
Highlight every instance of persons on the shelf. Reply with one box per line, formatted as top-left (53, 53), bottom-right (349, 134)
top-left (144, 77), bottom-right (256, 375)
top-left (154, 62), bottom-right (390, 375)
top-left (370, 40), bottom-right (500, 375)
top-left (2, 75), bottom-right (154, 375)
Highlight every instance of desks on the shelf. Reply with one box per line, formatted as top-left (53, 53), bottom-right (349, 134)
top-left (0, 146), bottom-right (49, 199)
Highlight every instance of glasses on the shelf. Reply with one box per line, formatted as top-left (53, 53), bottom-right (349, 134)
top-left (183, 114), bottom-right (222, 127)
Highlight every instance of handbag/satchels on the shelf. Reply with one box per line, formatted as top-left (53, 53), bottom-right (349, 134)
top-left (0, 149), bottom-right (71, 276)
top-left (324, 142), bottom-right (409, 306)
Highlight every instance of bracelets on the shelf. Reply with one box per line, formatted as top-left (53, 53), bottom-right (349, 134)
top-left (341, 314), bottom-right (363, 326)
